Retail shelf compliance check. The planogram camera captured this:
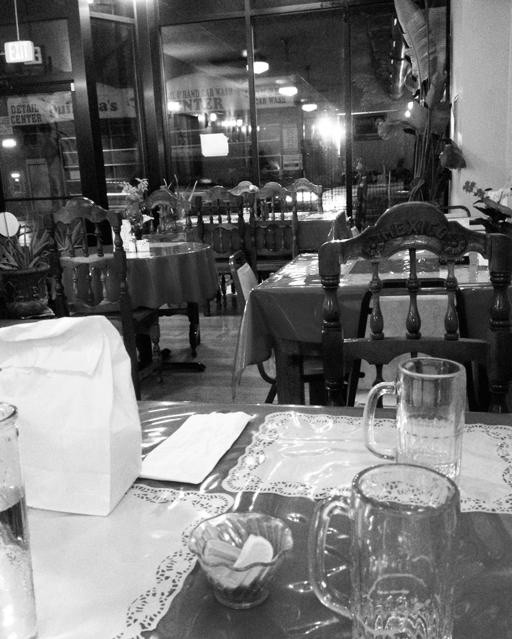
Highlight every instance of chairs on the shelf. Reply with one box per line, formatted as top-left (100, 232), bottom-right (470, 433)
top-left (317, 202), bottom-right (511, 406)
top-left (52, 177), bottom-right (510, 316)
top-left (52, 194), bottom-right (159, 398)
top-left (225, 246), bottom-right (366, 408)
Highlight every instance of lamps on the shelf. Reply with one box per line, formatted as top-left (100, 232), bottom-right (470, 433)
top-left (300, 96), bottom-right (317, 111)
top-left (2, 0), bottom-right (33, 65)
top-left (278, 81), bottom-right (298, 96)
top-left (246, 53), bottom-right (270, 75)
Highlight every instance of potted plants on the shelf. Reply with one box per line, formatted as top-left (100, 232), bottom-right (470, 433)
top-left (0, 205), bottom-right (62, 320)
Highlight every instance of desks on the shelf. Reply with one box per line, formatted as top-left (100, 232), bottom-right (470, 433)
top-left (55, 242), bottom-right (220, 375)
top-left (229, 251), bottom-right (511, 408)
top-left (0, 401), bottom-right (511, 638)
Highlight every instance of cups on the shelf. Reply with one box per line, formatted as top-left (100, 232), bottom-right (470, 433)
top-left (361, 354), bottom-right (468, 488)
top-left (305, 462), bottom-right (463, 639)
top-left (187, 511), bottom-right (295, 610)
